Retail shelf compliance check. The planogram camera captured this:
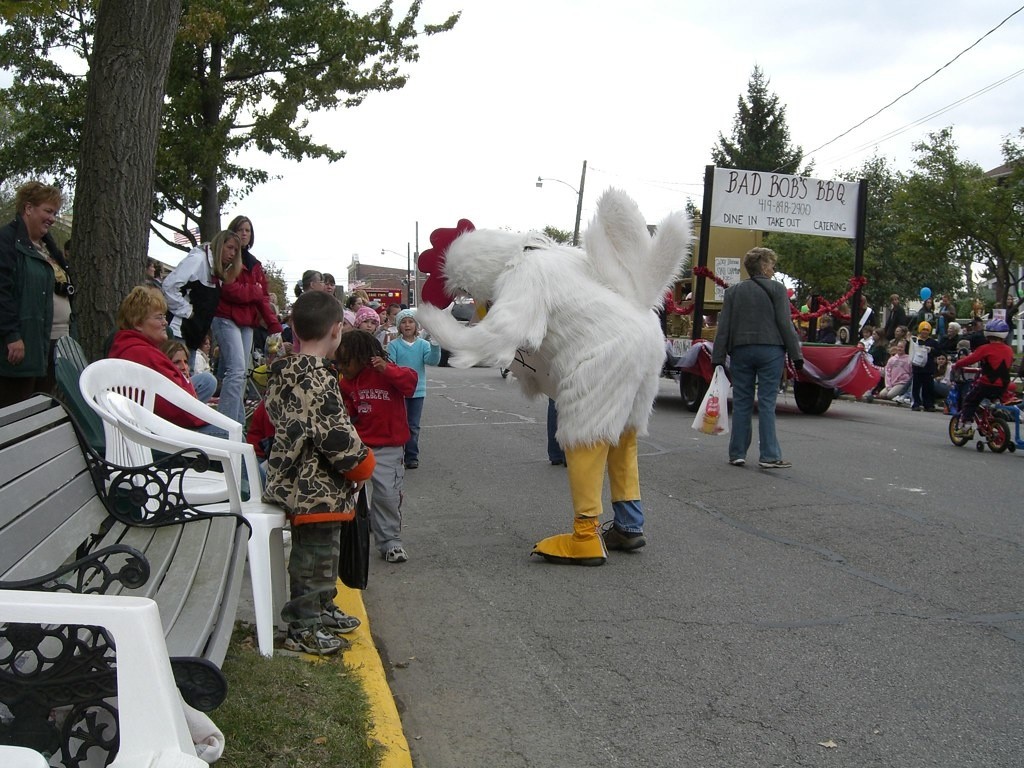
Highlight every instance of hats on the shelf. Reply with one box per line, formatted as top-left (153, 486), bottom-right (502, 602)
top-left (919, 321), bottom-right (931, 333)
top-left (355, 305), bottom-right (380, 326)
top-left (959, 339), bottom-right (970, 350)
top-left (396, 309), bottom-right (420, 329)
top-left (968, 316), bottom-right (983, 325)
top-left (958, 349), bottom-right (968, 357)
top-left (371, 301), bottom-right (386, 314)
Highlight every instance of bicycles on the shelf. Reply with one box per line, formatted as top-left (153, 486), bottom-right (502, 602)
top-left (948, 367), bottom-right (1016, 454)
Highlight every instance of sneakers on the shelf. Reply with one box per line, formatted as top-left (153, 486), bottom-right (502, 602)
top-left (729, 458), bottom-right (745, 464)
top-left (602, 527), bottom-right (646, 550)
top-left (955, 426), bottom-right (975, 436)
top-left (892, 394), bottom-right (905, 404)
top-left (380, 546), bottom-right (409, 562)
top-left (759, 459), bottom-right (792, 468)
top-left (285, 606), bottom-right (361, 657)
top-left (406, 460), bottom-right (418, 468)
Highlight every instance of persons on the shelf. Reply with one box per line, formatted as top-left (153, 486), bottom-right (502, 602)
top-left (162, 216), bottom-right (284, 423)
top-left (277, 270), bottom-right (441, 470)
top-left (264, 290), bottom-right (376, 655)
top-left (0, 182), bottom-right (75, 411)
top-left (547, 398), bottom-right (567, 468)
top-left (333, 329), bottom-right (418, 563)
top-left (800, 293), bottom-right (1019, 412)
top-left (951, 318), bottom-right (1014, 437)
top-left (63, 239), bottom-right (71, 262)
top-left (712, 247), bottom-right (804, 468)
top-left (143, 257), bottom-right (187, 324)
top-left (108, 286), bottom-right (266, 493)
top-left (414, 187), bottom-right (691, 566)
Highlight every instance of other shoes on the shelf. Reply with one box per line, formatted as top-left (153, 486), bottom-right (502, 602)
top-left (552, 460), bottom-right (568, 468)
top-left (912, 406), bottom-right (921, 411)
top-left (904, 399), bottom-right (910, 404)
top-left (924, 407), bottom-right (935, 412)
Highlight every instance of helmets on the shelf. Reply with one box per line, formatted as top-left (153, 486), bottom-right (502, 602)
top-left (984, 319), bottom-right (1009, 338)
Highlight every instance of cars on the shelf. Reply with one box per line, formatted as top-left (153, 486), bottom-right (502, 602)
top-left (443, 296), bottom-right (494, 328)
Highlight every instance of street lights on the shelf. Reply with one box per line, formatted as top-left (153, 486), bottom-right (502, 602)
top-left (535, 176), bottom-right (583, 248)
top-left (381, 248), bottom-right (420, 308)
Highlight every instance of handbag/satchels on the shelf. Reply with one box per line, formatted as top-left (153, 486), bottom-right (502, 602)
top-left (691, 365), bottom-right (731, 436)
top-left (264, 333), bottom-right (286, 361)
top-left (909, 337), bottom-right (928, 368)
top-left (338, 483), bottom-right (372, 591)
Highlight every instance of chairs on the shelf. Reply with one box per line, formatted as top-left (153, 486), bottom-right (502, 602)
top-left (73, 356), bottom-right (289, 660)
top-left (2, 588), bottom-right (227, 764)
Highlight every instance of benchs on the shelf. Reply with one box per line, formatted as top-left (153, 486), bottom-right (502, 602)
top-left (0, 373), bottom-right (251, 768)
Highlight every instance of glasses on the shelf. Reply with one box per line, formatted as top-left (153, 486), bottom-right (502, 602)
top-left (770, 265), bottom-right (776, 270)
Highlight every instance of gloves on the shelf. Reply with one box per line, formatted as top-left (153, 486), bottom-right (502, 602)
top-left (711, 363), bottom-right (724, 372)
top-left (793, 359), bottom-right (804, 370)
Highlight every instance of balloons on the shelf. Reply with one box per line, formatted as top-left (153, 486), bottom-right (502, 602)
top-left (1018, 289), bottom-right (1024, 297)
top-left (920, 287), bottom-right (931, 301)
